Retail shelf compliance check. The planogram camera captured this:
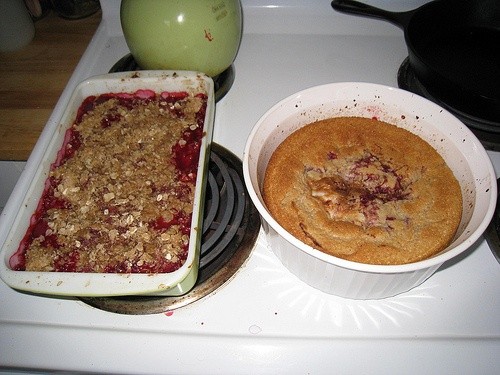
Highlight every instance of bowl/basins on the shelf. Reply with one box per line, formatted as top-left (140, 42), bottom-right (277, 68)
top-left (242, 82), bottom-right (498, 300)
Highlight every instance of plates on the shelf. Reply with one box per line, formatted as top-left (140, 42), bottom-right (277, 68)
top-left (1, 71), bottom-right (214, 296)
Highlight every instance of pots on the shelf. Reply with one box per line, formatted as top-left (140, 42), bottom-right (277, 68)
top-left (330, 0), bottom-right (500, 108)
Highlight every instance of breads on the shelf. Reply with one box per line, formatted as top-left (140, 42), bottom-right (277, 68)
top-left (263, 115), bottom-right (463, 266)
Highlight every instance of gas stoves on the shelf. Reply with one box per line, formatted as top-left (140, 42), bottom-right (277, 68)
top-left (0, 1), bottom-right (499, 374)
